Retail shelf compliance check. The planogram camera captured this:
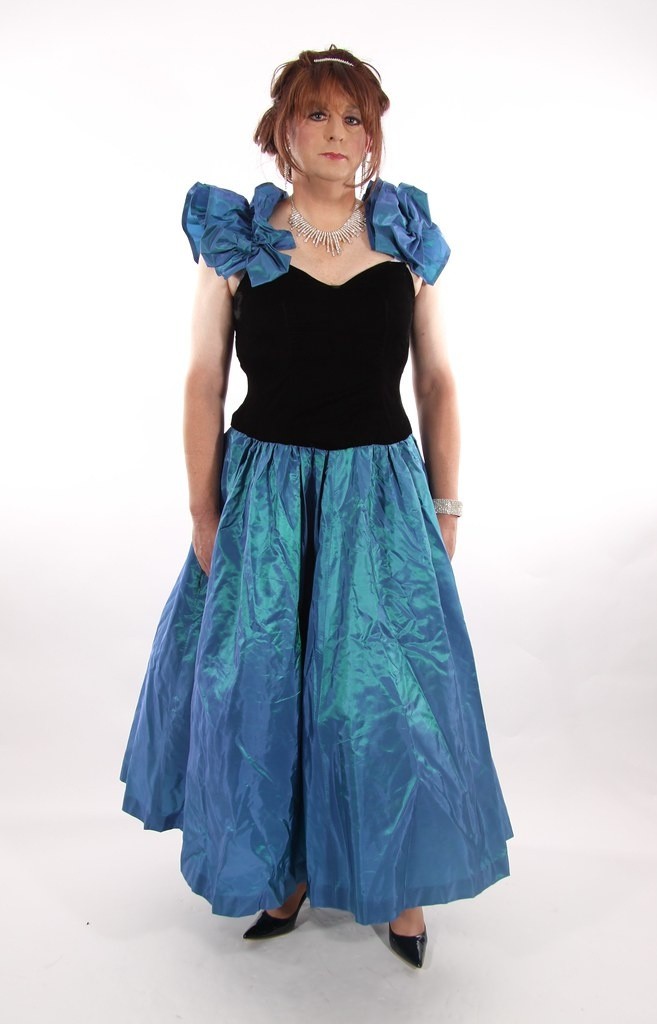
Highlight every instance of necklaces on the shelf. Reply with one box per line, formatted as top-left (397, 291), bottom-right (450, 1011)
top-left (284, 195), bottom-right (368, 260)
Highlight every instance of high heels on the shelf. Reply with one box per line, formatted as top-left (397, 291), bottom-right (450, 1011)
top-left (387, 921), bottom-right (432, 970)
top-left (244, 883), bottom-right (308, 940)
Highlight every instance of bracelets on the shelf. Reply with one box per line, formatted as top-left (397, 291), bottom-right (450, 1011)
top-left (433, 496), bottom-right (465, 521)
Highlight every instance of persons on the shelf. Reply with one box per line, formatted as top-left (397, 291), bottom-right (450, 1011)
top-left (115, 40), bottom-right (514, 977)
